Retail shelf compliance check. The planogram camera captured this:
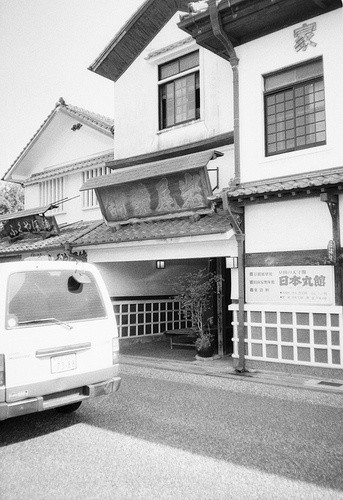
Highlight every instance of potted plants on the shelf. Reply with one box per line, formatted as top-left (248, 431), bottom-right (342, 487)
top-left (168, 267), bottom-right (226, 356)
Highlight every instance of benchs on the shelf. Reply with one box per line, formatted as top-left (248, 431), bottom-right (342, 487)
top-left (165, 328), bottom-right (196, 349)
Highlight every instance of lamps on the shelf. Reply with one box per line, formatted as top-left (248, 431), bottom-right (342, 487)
top-left (156, 260), bottom-right (165, 269)
top-left (225, 257), bottom-right (238, 269)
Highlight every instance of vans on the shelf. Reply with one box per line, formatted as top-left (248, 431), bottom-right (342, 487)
top-left (0, 259), bottom-right (122, 427)
